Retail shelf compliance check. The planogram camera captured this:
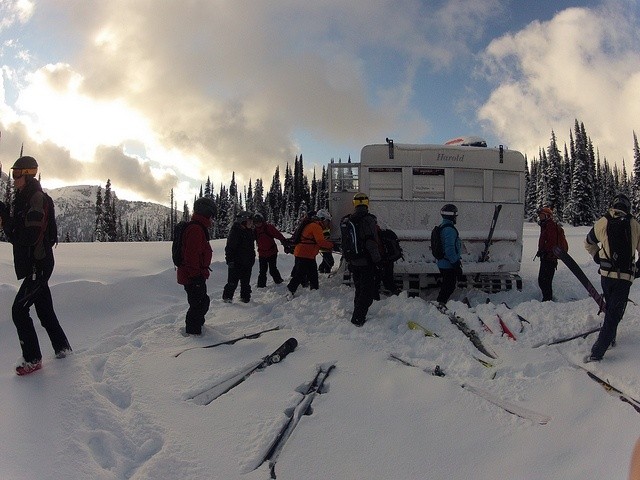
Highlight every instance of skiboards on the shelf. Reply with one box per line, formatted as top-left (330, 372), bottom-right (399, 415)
top-left (537, 326), bottom-right (601, 347)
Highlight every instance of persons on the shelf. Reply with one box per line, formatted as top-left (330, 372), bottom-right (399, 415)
top-left (0, 156), bottom-right (73, 376)
top-left (306, 212), bottom-right (334, 274)
top-left (252, 213), bottom-right (286, 288)
top-left (536, 208), bottom-right (558, 302)
top-left (342, 193), bottom-right (382, 326)
top-left (286, 210), bottom-right (332, 297)
top-left (222, 212), bottom-right (256, 303)
top-left (436, 204), bottom-right (463, 309)
top-left (583, 194), bottom-right (640, 362)
top-left (177, 198), bottom-right (218, 336)
top-left (371, 214), bottom-right (401, 301)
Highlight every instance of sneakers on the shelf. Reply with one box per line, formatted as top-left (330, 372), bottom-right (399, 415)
top-left (16, 359), bottom-right (43, 376)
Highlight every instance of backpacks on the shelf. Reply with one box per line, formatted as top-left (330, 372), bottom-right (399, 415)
top-left (44, 193), bottom-right (57, 247)
top-left (431, 223), bottom-right (459, 260)
top-left (284, 218), bottom-right (319, 254)
top-left (544, 221), bottom-right (568, 253)
top-left (602, 212), bottom-right (634, 269)
top-left (340, 214), bottom-right (377, 260)
top-left (172, 221), bottom-right (209, 268)
top-left (377, 229), bottom-right (403, 265)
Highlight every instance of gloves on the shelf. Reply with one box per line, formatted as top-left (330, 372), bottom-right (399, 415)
top-left (455, 265), bottom-right (462, 276)
top-left (0, 201), bottom-right (11, 225)
top-left (191, 280), bottom-right (201, 297)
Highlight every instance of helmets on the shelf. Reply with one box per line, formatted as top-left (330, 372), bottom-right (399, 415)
top-left (440, 204), bottom-right (458, 216)
top-left (537, 208), bottom-right (552, 218)
top-left (252, 213), bottom-right (264, 221)
top-left (613, 194), bottom-right (631, 209)
top-left (194, 199), bottom-right (217, 216)
top-left (316, 209), bottom-right (332, 222)
top-left (352, 192), bottom-right (368, 208)
top-left (11, 156), bottom-right (38, 169)
top-left (237, 212), bottom-right (254, 225)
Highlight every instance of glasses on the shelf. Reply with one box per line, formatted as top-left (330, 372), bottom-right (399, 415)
top-left (253, 218), bottom-right (263, 223)
top-left (13, 168), bottom-right (37, 179)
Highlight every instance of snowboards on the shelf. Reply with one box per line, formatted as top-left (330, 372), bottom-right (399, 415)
top-left (478, 204), bottom-right (503, 262)
top-left (240, 365), bottom-right (338, 480)
top-left (486, 297), bottom-right (518, 341)
top-left (174, 325), bottom-right (280, 358)
top-left (183, 338), bottom-right (298, 405)
top-left (560, 253), bottom-right (607, 313)
top-left (406, 320), bottom-right (492, 370)
top-left (384, 352), bottom-right (550, 426)
top-left (501, 301), bottom-right (530, 333)
top-left (569, 364), bottom-right (640, 412)
top-left (462, 296), bottom-right (494, 334)
